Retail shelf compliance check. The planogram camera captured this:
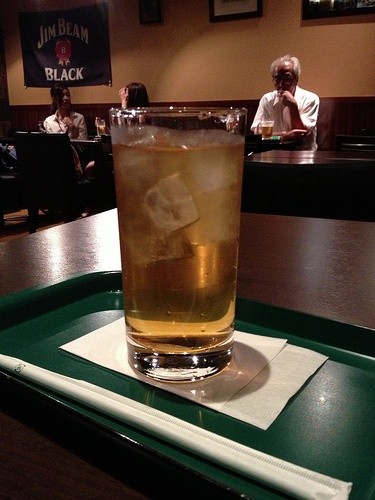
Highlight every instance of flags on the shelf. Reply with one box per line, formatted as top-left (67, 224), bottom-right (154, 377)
top-left (17, 3), bottom-right (112, 88)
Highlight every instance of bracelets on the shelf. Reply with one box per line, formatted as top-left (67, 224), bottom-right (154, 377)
top-left (121, 107), bottom-right (127, 109)
top-left (68, 122), bottom-right (72, 125)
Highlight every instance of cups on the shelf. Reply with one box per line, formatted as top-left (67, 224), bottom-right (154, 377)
top-left (39, 121), bottom-right (47, 133)
top-left (96, 119), bottom-right (106, 138)
top-left (108, 105), bottom-right (249, 383)
top-left (260, 121), bottom-right (274, 140)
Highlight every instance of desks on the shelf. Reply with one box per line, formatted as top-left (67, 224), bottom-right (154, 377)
top-left (244, 149), bottom-right (375, 169)
top-left (0, 206), bottom-right (375, 500)
top-left (70, 138), bottom-right (100, 147)
top-left (246, 137), bottom-right (299, 158)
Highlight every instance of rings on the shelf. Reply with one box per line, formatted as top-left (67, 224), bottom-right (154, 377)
top-left (281, 94), bottom-right (282, 97)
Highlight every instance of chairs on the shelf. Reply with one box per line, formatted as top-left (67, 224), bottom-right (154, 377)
top-left (333, 134), bottom-right (375, 151)
top-left (13, 131), bottom-right (111, 236)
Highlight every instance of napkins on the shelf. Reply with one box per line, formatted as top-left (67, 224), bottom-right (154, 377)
top-left (57, 313), bottom-right (331, 431)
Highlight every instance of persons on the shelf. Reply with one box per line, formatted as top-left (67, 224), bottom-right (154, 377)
top-left (250, 55), bottom-right (320, 151)
top-left (43, 83), bottom-right (88, 140)
top-left (119, 82), bottom-right (150, 109)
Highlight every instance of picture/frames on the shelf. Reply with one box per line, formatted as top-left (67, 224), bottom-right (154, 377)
top-left (208, 0), bottom-right (263, 23)
top-left (302, 0), bottom-right (375, 21)
top-left (138, 0), bottom-right (162, 25)
